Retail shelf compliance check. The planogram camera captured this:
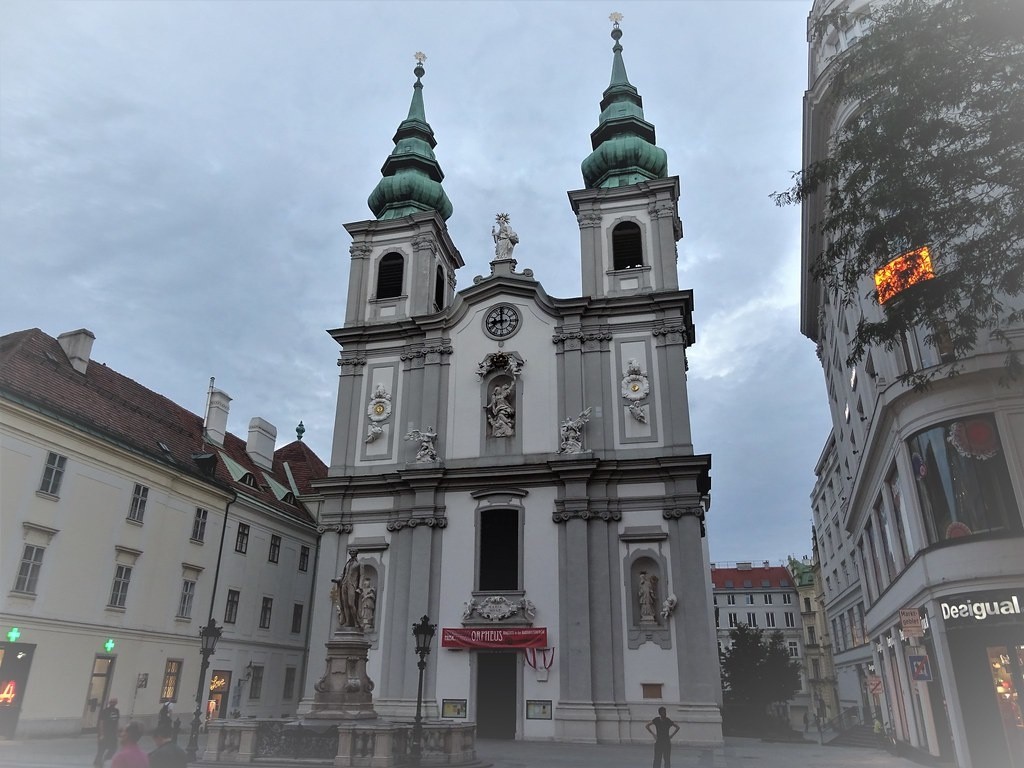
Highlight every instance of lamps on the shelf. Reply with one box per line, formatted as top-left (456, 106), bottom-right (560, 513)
top-left (238, 661), bottom-right (255, 686)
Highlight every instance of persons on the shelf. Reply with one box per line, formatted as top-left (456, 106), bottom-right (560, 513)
top-left (365, 422), bottom-right (382, 443)
top-left (803, 712), bottom-right (809, 733)
top-left (93, 697), bottom-right (120, 768)
top-left (638, 570), bottom-right (656, 616)
top-left (147, 726), bottom-right (188, 768)
top-left (518, 597), bottom-right (535, 621)
top-left (629, 401), bottom-right (647, 423)
top-left (661, 596), bottom-right (675, 619)
top-left (462, 598), bottom-right (477, 620)
top-left (813, 714), bottom-right (821, 732)
top-left (330, 547), bottom-right (375, 629)
top-left (872, 718), bottom-right (883, 750)
top-left (492, 220), bottom-right (519, 260)
top-left (561, 417), bottom-right (582, 444)
top-left (483, 358), bottom-right (520, 427)
top-left (645, 707), bottom-right (680, 768)
top-left (415, 426), bottom-right (438, 459)
top-left (105, 720), bottom-right (149, 768)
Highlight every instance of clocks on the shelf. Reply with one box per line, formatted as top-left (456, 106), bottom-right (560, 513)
top-left (481, 302), bottom-right (523, 341)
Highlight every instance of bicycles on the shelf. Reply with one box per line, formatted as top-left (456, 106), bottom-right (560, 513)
top-left (879, 722), bottom-right (899, 757)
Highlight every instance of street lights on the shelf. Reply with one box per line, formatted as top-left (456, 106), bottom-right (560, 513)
top-left (408, 615), bottom-right (437, 768)
top-left (186, 618), bottom-right (224, 762)
